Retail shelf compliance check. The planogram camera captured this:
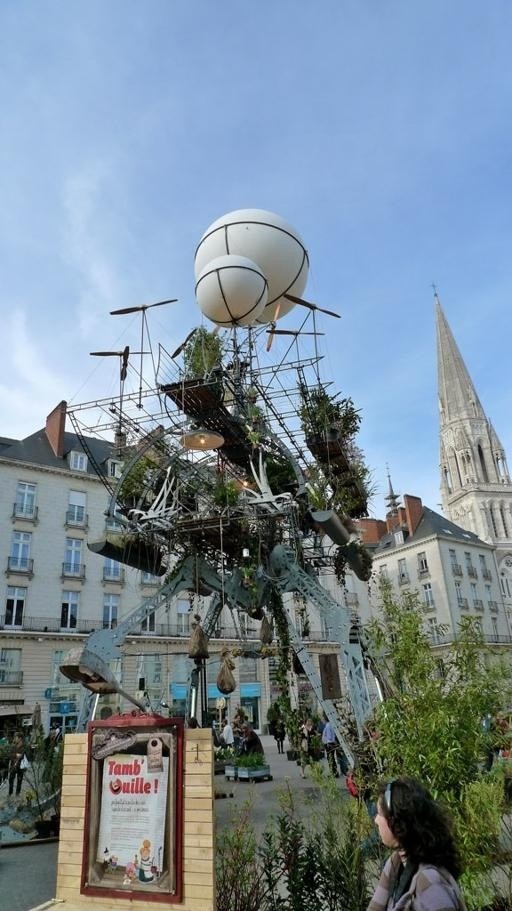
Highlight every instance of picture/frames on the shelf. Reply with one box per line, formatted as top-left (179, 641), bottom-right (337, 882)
top-left (83, 716), bottom-right (183, 907)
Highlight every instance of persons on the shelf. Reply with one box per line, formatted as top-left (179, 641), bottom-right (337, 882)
top-left (296, 717), bottom-right (318, 780)
top-left (364, 778), bottom-right (465, 911)
top-left (8, 732), bottom-right (26, 798)
top-left (322, 722), bottom-right (347, 779)
top-left (189, 713), bottom-right (265, 760)
top-left (273, 721), bottom-right (287, 753)
top-left (41, 731), bottom-right (56, 779)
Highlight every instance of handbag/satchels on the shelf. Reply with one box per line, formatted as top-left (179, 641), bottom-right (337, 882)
top-left (20, 755), bottom-right (28, 769)
